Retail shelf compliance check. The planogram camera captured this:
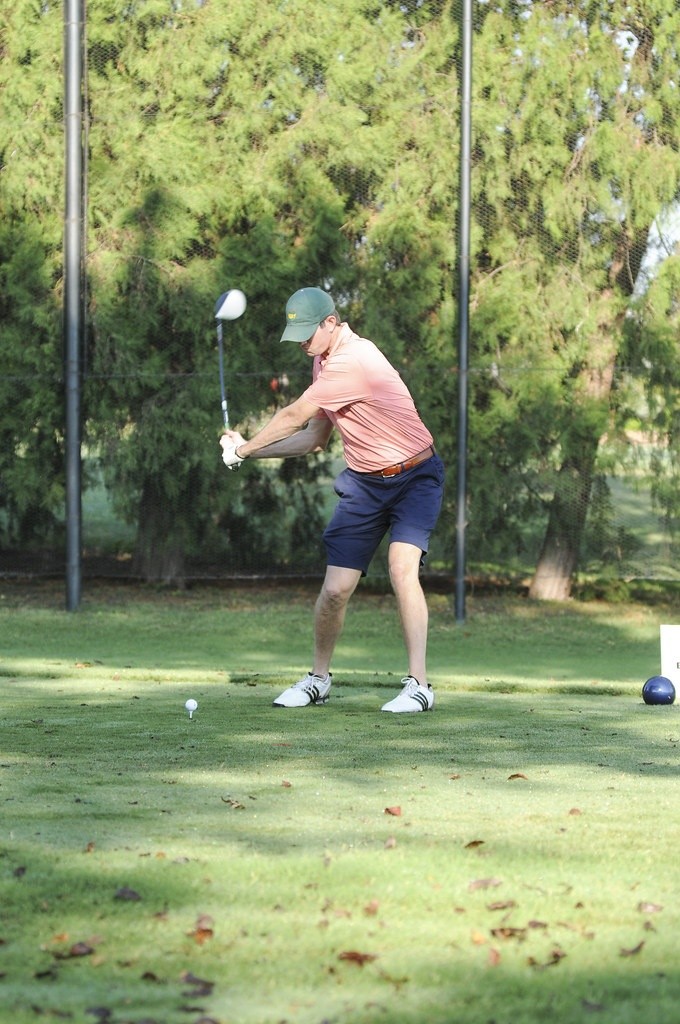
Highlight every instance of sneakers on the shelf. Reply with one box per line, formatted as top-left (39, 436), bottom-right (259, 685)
top-left (273, 673), bottom-right (332, 708)
top-left (380, 677), bottom-right (434, 712)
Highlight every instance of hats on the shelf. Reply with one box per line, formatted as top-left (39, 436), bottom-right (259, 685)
top-left (280, 287), bottom-right (335, 342)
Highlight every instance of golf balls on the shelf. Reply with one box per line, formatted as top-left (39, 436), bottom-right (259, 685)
top-left (186, 699), bottom-right (197, 711)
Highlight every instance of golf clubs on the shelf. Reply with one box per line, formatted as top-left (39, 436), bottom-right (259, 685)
top-left (212, 289), bottom-right (248, 472)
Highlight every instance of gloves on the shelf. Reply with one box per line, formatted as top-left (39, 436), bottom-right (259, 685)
top-left (222, 442), bottom-right (246, 471)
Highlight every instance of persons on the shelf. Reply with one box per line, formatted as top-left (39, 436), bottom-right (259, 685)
top-left (221, 287), bottom-right (447, 713)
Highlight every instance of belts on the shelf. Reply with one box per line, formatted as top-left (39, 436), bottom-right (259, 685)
top-left (362, 444), bottom-right (436, 478)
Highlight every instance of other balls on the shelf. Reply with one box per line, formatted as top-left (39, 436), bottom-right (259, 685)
top-left (642, 675), bottom-right (676, 707)
